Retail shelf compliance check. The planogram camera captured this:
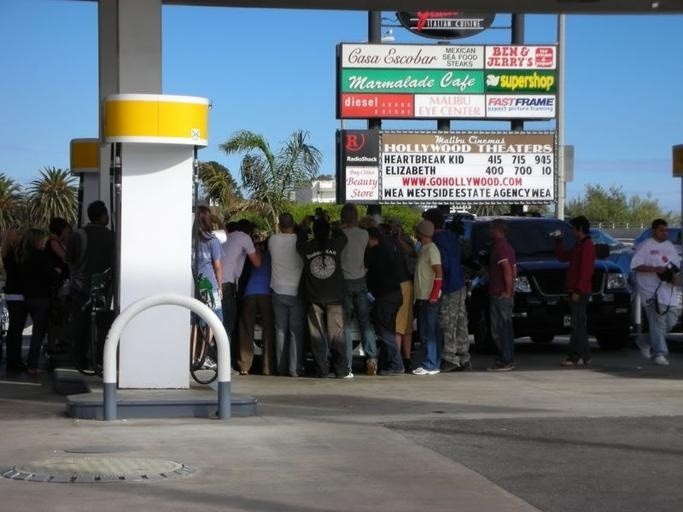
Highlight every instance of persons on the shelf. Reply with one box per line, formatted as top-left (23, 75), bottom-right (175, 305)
top-left (488, 219), bottom-right (517, 371)
top-left (65, 201), bottom-right (116, 372)
top-left (268, 212), bottom-right (309, 377)
top-left (379, 224), bottom-right (415, 371)
top-left (330, 203), bottom-right (380, 375)
top-left (192, 206), bottom-right (225, 371)
top-left (548, 217), bottom-right (596, 367)
top-left (411, 222), bottom-right (443, 376)
top-left (237, 237), bottom-right (276, 375)
top-left (629, 219), bottom-right (682, 366)
top-left (222, 219), bottom-right (262, 376)
top-left (24, 228), bottom-right (62, 376)
top-left (423, 208), bottom-right (472, 372)
top-left (297, 214), bottom-right (354, 378)
top-left (45, 217), bottom-right (73, 375)
top-left (359, 217), bottom-right (405, 374)
top-left (0, 225), bottom-right (27, 374)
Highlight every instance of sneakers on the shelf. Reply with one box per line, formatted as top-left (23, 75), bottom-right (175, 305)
top-left (198, 356), bottom-right (218, 370)
top-left (413, 366), bottom-right (440, 375)
top-left (578, 359), bottom-right (591, 365)
top-left (652, 355), bottom-right (671, 366)
top-left (229, 367), bottom-right (238, 376)
top-left (487, 360), bottom-right (515, 371)
top-left (635, 337), bottom-right (651, 361)
top-left (366, 356), bottom-right (378, 375)
top-left (560, 356), bottom-right (582, 367)
top-left (380, 362), bottom-right (404, 375)
top-left (335, 367), bottom-right (355, 378)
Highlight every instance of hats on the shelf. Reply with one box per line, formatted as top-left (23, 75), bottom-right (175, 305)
top-left (360, 217), bottom-right (379, 229)
top-left (416, 221), bottom-right (435, 236)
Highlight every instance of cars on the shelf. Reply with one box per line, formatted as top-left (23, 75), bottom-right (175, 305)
top-left (587, 224), bottom-right (636, 296)
top-left (252, 306), bottom-right (383, 374)
top-left (636, 223), bottom-right (682, 256)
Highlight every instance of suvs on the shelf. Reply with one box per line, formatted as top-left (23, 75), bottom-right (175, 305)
top-left (448, 211), bottom-right (634, 354)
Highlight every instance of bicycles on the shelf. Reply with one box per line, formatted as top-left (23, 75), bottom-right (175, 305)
top-left (67, 264), bottom-right (118, 375)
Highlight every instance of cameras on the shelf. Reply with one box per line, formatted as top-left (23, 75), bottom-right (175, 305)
top-left (309, 207), bottom-right (330, 222)
top-left (655, 261), bottom-right (679, 283)
top-left (543, 229), bottom-right (567, 240)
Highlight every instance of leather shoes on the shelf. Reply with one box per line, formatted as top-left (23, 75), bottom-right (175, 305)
top-left (7, 364), bottom-right (26, 376)
top-left (24, 367), bottom-right (47, 377)
top-left (439, 361), bottom-right (459, 372)
